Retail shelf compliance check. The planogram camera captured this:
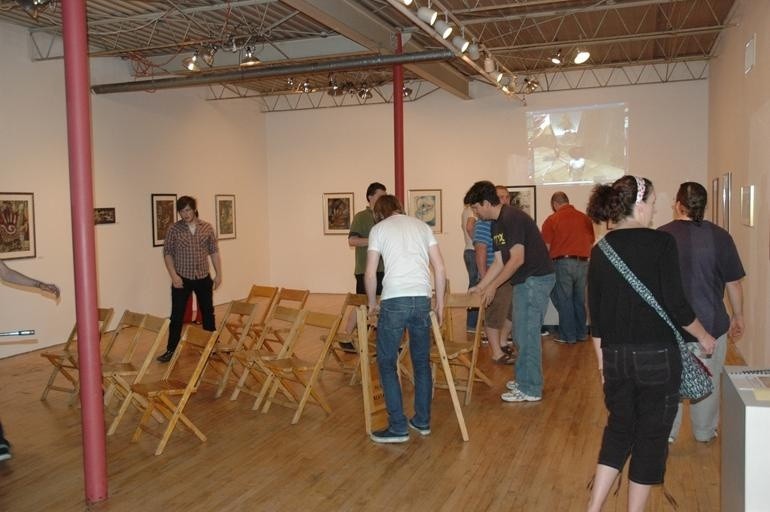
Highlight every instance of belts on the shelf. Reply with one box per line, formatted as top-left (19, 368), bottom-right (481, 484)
top-left (553, 255), bottom-right (590, 262)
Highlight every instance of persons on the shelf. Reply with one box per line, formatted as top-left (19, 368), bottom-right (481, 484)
top-left (337, 182), bottom-right (388, 354)
top-left (654, 182), bottom-right (745, 445)
top-left (583, 174), bottom-right (717, 512)
top-left (472, 184), bottom-right (521, 365)
top-left (463, 179), bottom-right (556, 401)
top-left (461, 195), bottom-right (486, 335)
top-left (0, 253), bottom-right (60, 461)
top-left (364, 194), bottom-right (446, 443)
top-left (156, 196), bottom-right (231, 363)
top-left (540, 192), bottom-right (595, 344)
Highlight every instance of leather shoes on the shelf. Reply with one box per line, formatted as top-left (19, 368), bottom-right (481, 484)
top-left (157, 352), bottom-right (172, 362)
top-left (338, 341), bottom-right (357, 354)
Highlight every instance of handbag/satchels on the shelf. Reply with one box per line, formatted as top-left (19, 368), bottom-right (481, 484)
top-left (674, 332), bottom-right (715, 399)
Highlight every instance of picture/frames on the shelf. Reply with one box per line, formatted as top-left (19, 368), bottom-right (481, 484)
top-left (215, 194), bottom-right (236, 240)
top-left (94, 208), bottom-right (115, 225)
top-left (720, 171), bottom-right (732, 233)
top-left (408, 189), bottom-right (443, 234)
top-left (0, 192), bottom-right (37, 260)
top-left (151, 193), bottom-right (178, 247)
top-left (505, 185), bottom-right (536, 224)
top-left (739, 183), bottom-right (753, 227)
top-left (321, 191), bottom-right (354, 235)
top-left (712, 178), bottom-right (719, 224)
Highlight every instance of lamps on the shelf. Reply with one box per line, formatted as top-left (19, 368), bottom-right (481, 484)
top-left (400, 0), bottom-right (540, 95)
top-left (571, 45), bottom-right (591, 65)
top-left (181, 45), bottom-right (413, 99)
top-left (551, 48), bottom-right (562, 65)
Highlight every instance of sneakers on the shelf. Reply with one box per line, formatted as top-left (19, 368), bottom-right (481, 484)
top-left (370, 428), bottom-right (410, 443)
top-left (408, 418), bottom-right (432, 435)
top-left (480, 329), bottom-right (589, 402)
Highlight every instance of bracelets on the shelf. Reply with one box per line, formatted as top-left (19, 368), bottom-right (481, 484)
top-left (36, 281), bottom-right (45, 289)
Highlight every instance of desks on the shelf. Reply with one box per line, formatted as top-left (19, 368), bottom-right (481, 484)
top-left (720, 364), bottom-right (769, 511)
